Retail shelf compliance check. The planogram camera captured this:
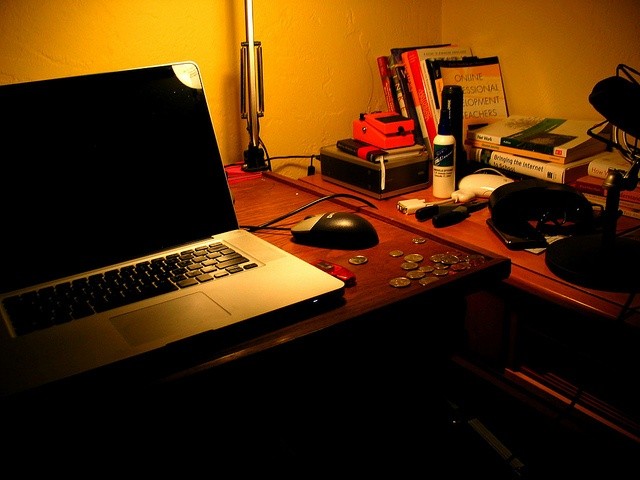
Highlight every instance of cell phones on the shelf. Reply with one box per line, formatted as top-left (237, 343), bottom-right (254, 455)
top-left (486, 217), bottom-right (547, 249)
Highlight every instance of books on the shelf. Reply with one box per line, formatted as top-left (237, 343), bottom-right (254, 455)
top-left (377, 42), bottom-right (509, 163)
top-left (463, 137), bottom-right (607, 165)
top-left (465, 147), bottom-right (610, 185)
top-left (466, 113), bottom-right (609, 158)
top-left (573, 153), bottom-right (640, 219)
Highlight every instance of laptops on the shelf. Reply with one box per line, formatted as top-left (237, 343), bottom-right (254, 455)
top-left (0, 60), bottom-right (346, 408)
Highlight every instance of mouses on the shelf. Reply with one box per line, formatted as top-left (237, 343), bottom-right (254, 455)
top-left (291, 210), bottom-right (379, 249)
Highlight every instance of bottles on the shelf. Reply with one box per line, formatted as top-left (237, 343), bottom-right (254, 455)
top-left (433, 108), bottom-right (455, 198)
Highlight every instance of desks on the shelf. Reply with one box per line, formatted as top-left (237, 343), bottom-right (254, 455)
top-left (0, 165), bottom-right (512, 477)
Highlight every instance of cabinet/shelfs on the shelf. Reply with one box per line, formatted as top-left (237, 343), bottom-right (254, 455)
top-left (296, 166), bottom-right (640, 478)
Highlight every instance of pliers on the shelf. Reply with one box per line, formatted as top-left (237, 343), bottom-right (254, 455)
top-left (415, 198), bottom-right (488, 228)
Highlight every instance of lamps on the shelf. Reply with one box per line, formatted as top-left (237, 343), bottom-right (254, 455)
top-left (544, 62), bottom-right (640, 293)
